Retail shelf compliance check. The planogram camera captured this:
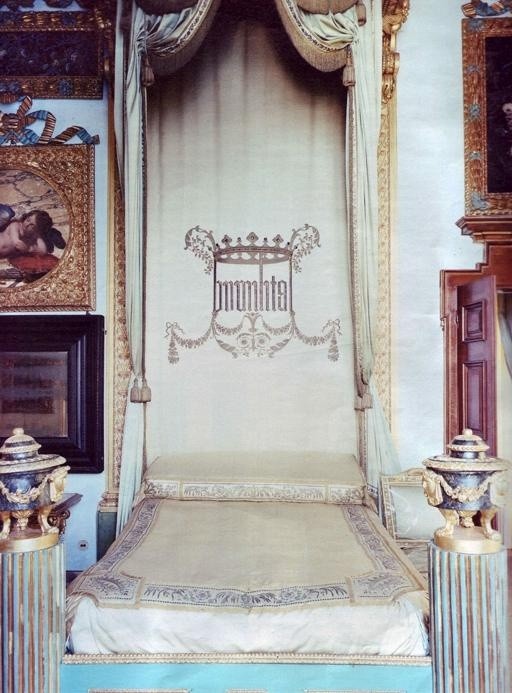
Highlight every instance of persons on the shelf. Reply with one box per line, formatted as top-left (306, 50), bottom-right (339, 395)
top-left (0, 210), bottom-right (53, 258)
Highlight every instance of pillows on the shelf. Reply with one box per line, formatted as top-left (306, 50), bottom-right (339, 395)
top-left (130, 450), bottom-right (369, 510)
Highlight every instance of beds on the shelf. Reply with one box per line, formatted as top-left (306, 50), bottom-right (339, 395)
top-left (61, 452), bottom-right (435, 691)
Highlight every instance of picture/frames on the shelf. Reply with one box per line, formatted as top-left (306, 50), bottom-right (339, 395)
top-left (1, 312), bottom-right (107, 475)
top-left (1, 140), bottom-right (98, 312)
top-left (460, 12), bottom-right (511, 219)
top-left (378, 472), bottom-right (433, 550)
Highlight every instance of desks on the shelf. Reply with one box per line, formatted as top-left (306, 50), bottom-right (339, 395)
top-left (1, 490), bottom-right (85, 536)
